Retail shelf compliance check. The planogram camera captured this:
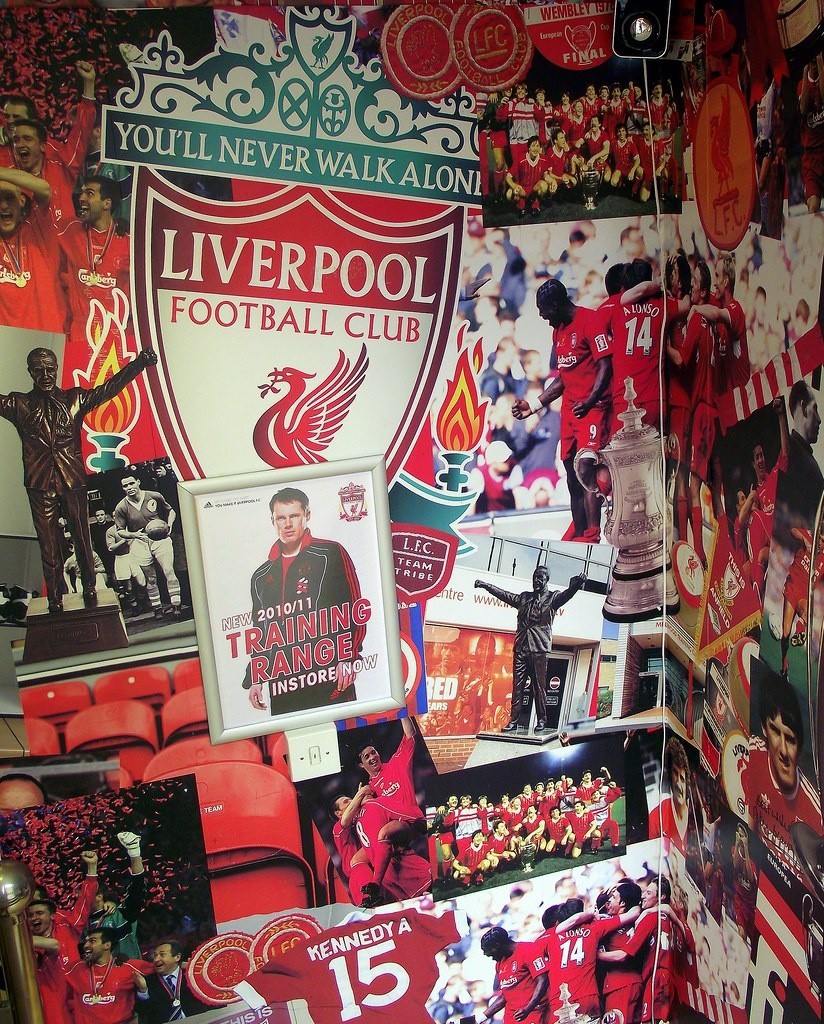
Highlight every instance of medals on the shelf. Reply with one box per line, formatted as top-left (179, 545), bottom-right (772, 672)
top-left (88, 275), bottom-right (100, 285)
top-left (15, 275), bottom-right (28, 289)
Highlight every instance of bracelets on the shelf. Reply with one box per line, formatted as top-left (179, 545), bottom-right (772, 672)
top-left (526, 397), bottom-right (543, 414)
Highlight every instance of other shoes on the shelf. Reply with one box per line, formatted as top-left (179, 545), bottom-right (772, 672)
top-left (361, 882), bottom-right (380, 896)
top-left (153, 605), bottom-right (163, 620)
top-left (463, 883), bottom-right (471, 890)
top-left (132, 601), bottom-right (153, 617)
top-left (362, 894), bottom-right (381, 908)
top-left (612, 846), bottom-right (621, 853)
top-left (494, 192), bottom-right (577, 218)
top-left (592, 848), bottom-right (599, 855)
top-left (173, 605), bottom-right (182, 617)
top-left (476, 880), bottom-right (482, 886)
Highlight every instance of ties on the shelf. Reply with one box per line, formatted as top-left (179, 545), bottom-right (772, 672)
top-left (166, 975), bottom-right (177, 992)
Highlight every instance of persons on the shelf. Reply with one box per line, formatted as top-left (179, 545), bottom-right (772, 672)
top-left (0, 0), bottom-right (824, 1024)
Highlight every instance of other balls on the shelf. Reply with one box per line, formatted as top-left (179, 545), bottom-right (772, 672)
top-left (144, 518), bottom-right (170, 542)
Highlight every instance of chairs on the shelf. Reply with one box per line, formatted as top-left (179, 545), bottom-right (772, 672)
top-left (20, 657), bottom-right (352, 925)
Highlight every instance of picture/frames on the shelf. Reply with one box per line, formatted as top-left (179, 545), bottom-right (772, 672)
top-left (177, 454), bottom-right (407, 745)
